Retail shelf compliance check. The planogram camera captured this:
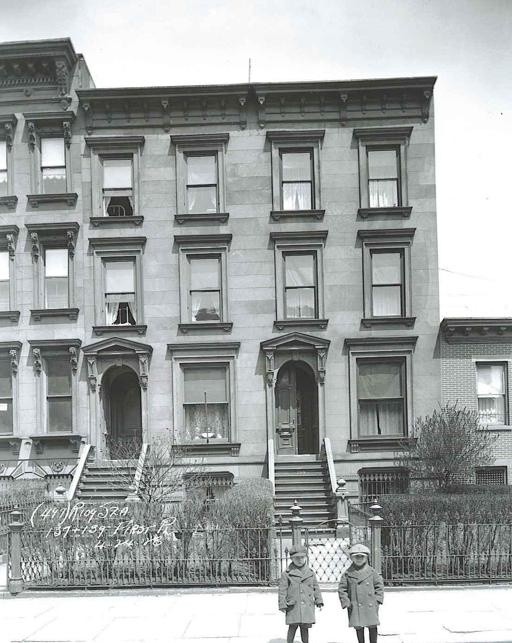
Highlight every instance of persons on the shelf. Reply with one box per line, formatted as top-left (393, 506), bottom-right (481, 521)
top-left (337, 542), bottom-right (387, 642)
top-left (278, 543), bottom-right (323, 643)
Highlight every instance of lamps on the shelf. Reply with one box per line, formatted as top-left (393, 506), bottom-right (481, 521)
top-left (87, 374), bottom-right (97, 391)
top-left (266, 368), bottom-right (274, 387)
top-left (139, 371), bottom-right (149, 389)
top-left (318, 366), bottom-right (327, 384)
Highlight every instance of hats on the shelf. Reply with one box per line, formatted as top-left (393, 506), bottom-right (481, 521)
top-left (348, 542), bottom-right (371, 557)
top-left (288, 544), bottom-right (308, 558)
